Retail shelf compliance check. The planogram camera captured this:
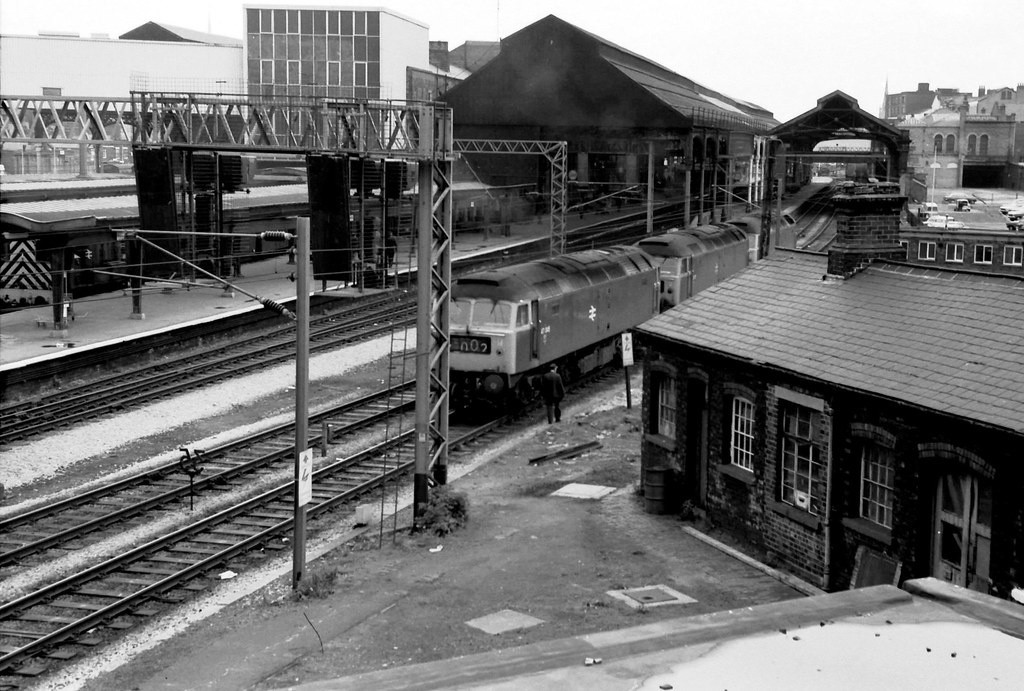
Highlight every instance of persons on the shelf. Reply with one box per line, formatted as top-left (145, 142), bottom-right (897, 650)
top-left (540, 364), bottom-right (564, 424)
top-left (384, 232), bottom-right (397, 267)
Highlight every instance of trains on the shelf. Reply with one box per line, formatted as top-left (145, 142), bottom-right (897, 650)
top-left (432, 221), bottom-right (747, 421)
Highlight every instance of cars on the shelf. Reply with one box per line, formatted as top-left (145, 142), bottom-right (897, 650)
top-left (920, 192), bottom-right (1024, 231)
